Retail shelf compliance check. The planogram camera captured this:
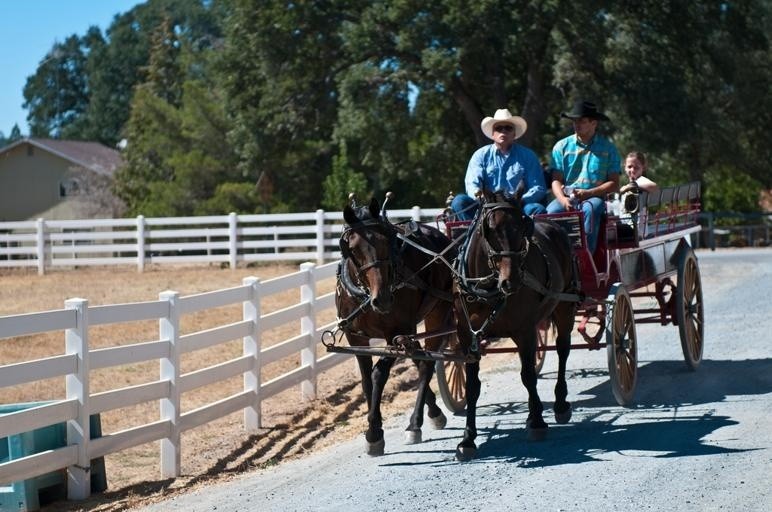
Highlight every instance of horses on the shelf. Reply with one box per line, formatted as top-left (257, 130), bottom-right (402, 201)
top-left (450, 176), bottom-right (583, 462)
top-left (333, 195), bottom-right (455, 457)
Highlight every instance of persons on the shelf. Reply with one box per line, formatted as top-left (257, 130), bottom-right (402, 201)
top-left (604, 151), bottom-right (658, 239)
top-left (546, 99), bottom-right (622, 255)
top-left (451, 108), bottom-right (547, 221)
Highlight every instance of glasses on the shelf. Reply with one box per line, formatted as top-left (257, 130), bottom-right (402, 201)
top-left (493, 125), bottom-right (513, 133)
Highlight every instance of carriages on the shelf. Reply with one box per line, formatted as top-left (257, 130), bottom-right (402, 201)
top-left (321, 180), bottom-right (706, 462)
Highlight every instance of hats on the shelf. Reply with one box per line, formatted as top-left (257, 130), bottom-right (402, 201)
top-left (561, 102), bottom-right (610, 122)
top-left (481, 108), bottom-right (527, 142)
